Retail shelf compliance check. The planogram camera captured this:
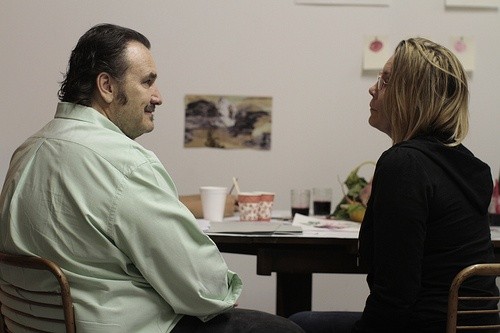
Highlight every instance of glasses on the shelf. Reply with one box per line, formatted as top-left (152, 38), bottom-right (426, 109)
top-left (376, 72), bottom-right (388, 91)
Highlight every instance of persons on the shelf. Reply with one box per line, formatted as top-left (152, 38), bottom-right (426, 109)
top-left (0, 23), bottom-right (304, 333)
top-left (290, 36), bottom-right (495, 333)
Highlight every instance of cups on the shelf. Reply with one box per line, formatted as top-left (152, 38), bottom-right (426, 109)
top-left (290, 189), bottom-right (311, 217)
top-left (312, 189), bottom-right (332, 218)
top-left (199, 186), bottom-right (228, 223)
top-left (238, 193), bottom-right (260, 221)
top-left (259, 193), bottom-right (275, 222)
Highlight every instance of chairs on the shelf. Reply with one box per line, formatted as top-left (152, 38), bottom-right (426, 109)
top-left (0, 252), bottom-right (76, 333)
top-left (448, 263), bottom-right (500, 333)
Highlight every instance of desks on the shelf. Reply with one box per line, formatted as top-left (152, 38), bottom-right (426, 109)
top-left (195, 210), bottom-right (500, 317)
